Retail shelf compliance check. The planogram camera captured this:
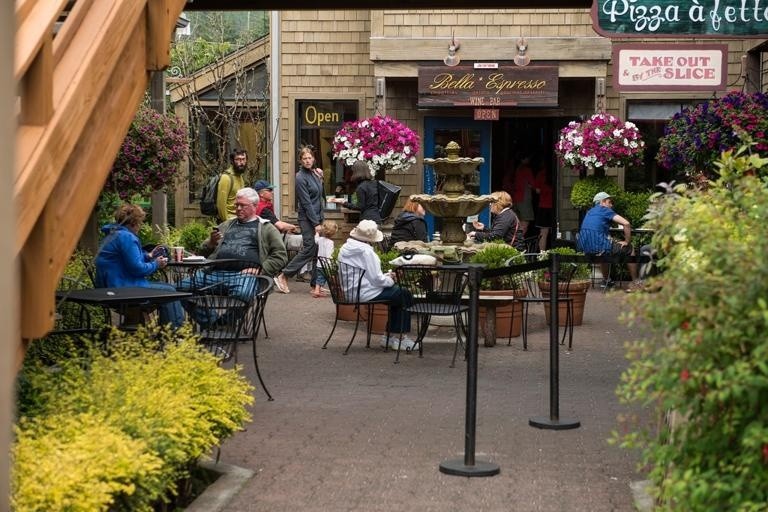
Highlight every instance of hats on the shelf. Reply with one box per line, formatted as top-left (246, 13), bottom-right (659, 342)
top-left (593, 192), bottom-right (616, 201)
top-left (350, 219), bottom-right (384, 242)
top-left (255, 180), bottom-right (274, 190)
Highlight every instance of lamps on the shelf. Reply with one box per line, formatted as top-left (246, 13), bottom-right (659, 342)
top-left (442, 29), bottom-right (461, 69)
top-left (512, 33), bottom-right (531, 69)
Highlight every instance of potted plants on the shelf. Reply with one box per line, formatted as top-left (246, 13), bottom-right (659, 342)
top-left (468, 244), bottom-right (532, 340)
top-left (330, 246), bottom-right (368, 322)
top-left (368, 248), bottom-right (405, 337)
top-left (537, 247), bottom-right (590, 326)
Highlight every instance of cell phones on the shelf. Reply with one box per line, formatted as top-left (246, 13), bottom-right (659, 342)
top-left (213, 226), bottom-right (219, 235)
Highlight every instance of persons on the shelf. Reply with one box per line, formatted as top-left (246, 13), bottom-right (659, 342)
top-left (578, 191), bottom-right (638, 283)
top-left (312, 219), bottom-right (339, 297)
top-left (529, 158), bottom-right (554, 254)
top-left (215, 148), bottom-right (248, 222)
top-left (94, 205), bottom-right (184, 334)
top-left (327, 160), bottom-right (382, 231)
top-left (254, 180), bottom-right (298, 233)
top-left (172, 187), bottom-right (289, 332)
top-left (386, 198), bottom-right (429, 253)
top-left (467, 191), bottom-right (524, 251)
top-left (511, 151), bottom-right (541, 239)
top-left (337, 218), bottom-right (419, 351)
top-left (273, 147), bottom-right (331, 293)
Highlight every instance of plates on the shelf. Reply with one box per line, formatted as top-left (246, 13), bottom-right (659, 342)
top-left (182, 258), bottom-right (203, 262)
top-left (327, 198), bottom-right (348, 203)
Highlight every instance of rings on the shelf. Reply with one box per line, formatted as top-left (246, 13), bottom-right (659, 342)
top-left (166, 258), bottom-right (167, 262)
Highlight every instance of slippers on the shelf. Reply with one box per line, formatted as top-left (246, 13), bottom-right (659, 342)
top-left (312, 287), bottom-right (331, 296)
top-left (274, 278), bottom-right (290, 293)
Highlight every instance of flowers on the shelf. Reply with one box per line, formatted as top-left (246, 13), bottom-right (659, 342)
top-left (326, 113), bottom-right (422, 180)
top-left (545, 110), bottom-right (651, 178)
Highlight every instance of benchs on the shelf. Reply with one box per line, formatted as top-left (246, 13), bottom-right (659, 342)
top-left (407, 294), bottom-right (513, 348)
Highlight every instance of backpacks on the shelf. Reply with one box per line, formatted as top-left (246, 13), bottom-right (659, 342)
top-left (200, 170), bottom-right (234, 216)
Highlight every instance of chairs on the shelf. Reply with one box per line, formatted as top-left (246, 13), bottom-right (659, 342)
top-left (514, 234), bottom-right (543, 265)
top-left (199, 275), bottom-right (278, 403)
top-left (319, 257), bottom-right (396, 356)
top-left (392, 267), bottom-right (470, 368)
top-left (188, 297), bottom-right (248, 371)
top-left (54, 296), bottom-right (92, 333)
top-left (507, 252), bottom-right (581, 351)
top-left (572, 227), bottom-right (624, 293)
top-left (84, 257), bottom-right (130, 327)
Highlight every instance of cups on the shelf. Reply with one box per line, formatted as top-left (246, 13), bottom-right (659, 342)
top-left (433, 234), bottom-right (440, 241)
top-left (466, 236), bottom-right (475, 241)
top-left (173, 247), bottom-right (184, 262)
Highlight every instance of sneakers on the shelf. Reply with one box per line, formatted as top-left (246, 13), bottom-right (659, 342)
top-left (381, 336), bottom-right (419, 350)
top-left (601, 281), bottom-right (615, 287)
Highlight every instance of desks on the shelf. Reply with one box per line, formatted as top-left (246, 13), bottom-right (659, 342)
top-left (163, 257), bottom-right (234, 324)
top-left (395, 260), bottom-right (488, 353)
top-left (607, 227), bottom-right (655, 252)
top-left (54, 288), bottom-right (192, 326)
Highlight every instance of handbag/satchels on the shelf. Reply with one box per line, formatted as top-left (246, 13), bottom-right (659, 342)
top-left (388, 250), bottom-right (436, 266)
top-left (142, 244), bottom-right (170, 262)
top-left (378, 180), bottom-right (401, 219)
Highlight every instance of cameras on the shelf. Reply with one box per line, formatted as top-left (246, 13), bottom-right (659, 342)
top-left (153, 246), bottom-right (171, 263)
